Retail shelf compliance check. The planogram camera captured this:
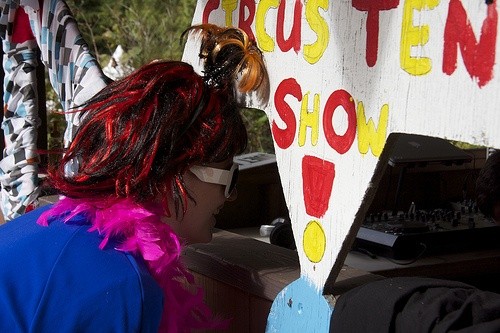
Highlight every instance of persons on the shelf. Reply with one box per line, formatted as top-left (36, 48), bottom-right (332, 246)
top-left (0, 23), bottom-right (271, 333)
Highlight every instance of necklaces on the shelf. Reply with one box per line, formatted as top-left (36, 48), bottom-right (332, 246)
top-left (36, 191), bottom-right (214, 333)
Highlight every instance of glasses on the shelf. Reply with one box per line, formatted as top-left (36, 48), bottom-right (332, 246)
top-left (185, 163), bottom-right (240, 198)
